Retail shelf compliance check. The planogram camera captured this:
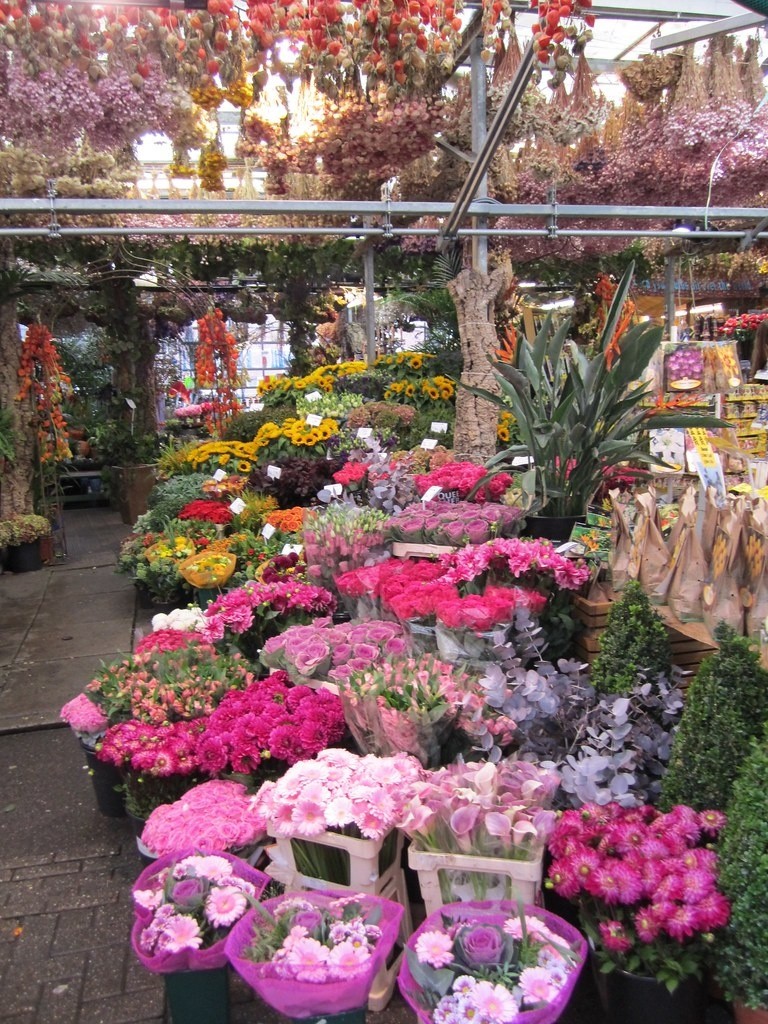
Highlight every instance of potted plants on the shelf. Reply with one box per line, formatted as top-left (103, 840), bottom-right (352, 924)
top-left (441, 258), bottom-right (738, 546)
top-left (84, 419), bottom-right (155, 527)
top-left (0, 514), bottom-right (53, 574)
top-left (39, 518), bottom-right (54, 562)
top-left (0, 521), bottom-right (15, 571)
top-left (712, 853), bottom-right (768, 1024)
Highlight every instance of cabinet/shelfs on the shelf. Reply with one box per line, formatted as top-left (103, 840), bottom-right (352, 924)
top-left (722, 395), bottom-right (768, 454)
top-left (663, 340), bottom-right (706, 392)
top-left (637, 392), bottom-right (721, 419)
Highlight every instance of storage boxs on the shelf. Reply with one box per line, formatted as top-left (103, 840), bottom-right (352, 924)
top-left (159, 966), bottom-right (230, 1024)
top-left (702, 340), bottom-right (716, 394)
top-left (709, 341), bottom-right (731, 391)
top-left (717, 340), bottom-right (745, 387)
top-left (277, 827), bottom-right (414, 1015)
top-left (406, 837), bottom-right (548, 917)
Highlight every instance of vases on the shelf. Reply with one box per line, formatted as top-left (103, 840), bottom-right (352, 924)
top-left (741, 330), bottom-right (756, 342)
top-left (79, 744), bottom-right (125, 819)
top-left (125, 809), bottom-right (155, 867)
top-left (587, 936), bottom-right (708, 1024)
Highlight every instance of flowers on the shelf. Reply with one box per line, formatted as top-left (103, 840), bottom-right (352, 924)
top-left (60, 312), bottom-right (768, 1024)
